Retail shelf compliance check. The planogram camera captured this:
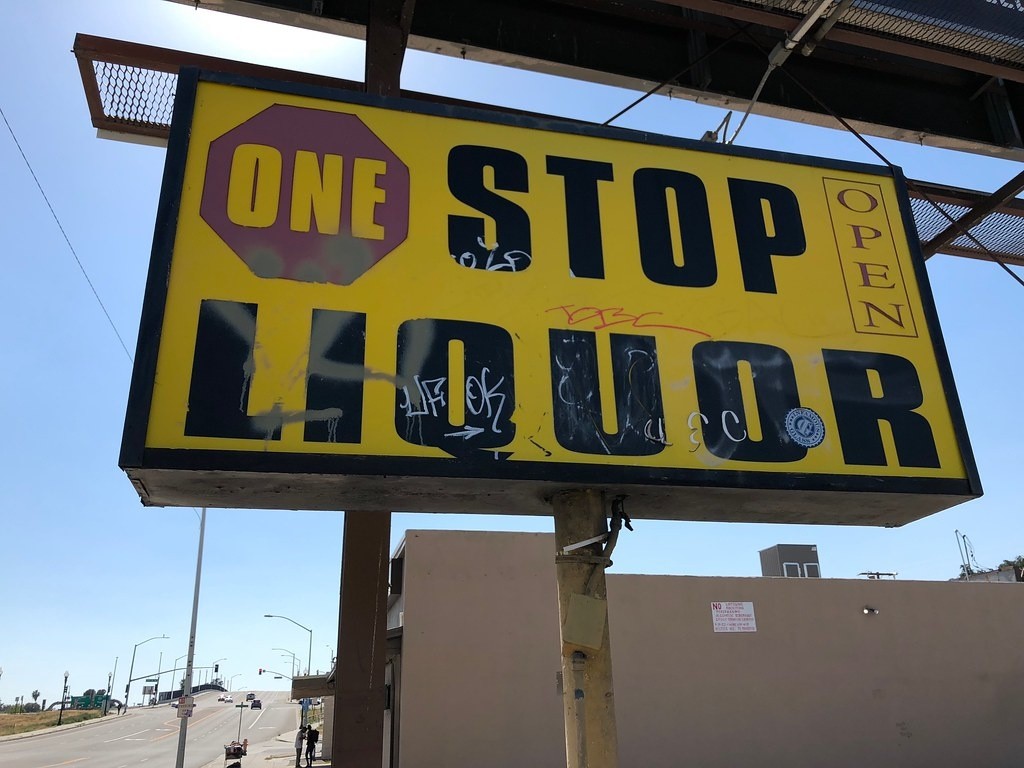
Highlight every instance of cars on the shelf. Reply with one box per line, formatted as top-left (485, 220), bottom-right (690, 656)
top-left (218, 694), bottom-right (233, 703)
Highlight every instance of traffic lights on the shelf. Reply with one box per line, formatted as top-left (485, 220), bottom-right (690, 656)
top-left (259, 669), bottom-right (262, 675)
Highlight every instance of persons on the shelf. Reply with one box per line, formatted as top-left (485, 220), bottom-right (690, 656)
top-left (118, 704), bottom-right (121, 715)
top-left (295, 727), bottom-right (308, 768)
top-left (305, 725), bottom-right (315, 768)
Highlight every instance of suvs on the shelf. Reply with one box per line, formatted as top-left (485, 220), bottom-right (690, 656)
top-left (246, 692), bottom-right (255, 700)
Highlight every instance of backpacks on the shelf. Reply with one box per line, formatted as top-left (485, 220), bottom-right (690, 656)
top-left (311, 730), bottom-right (318, 742)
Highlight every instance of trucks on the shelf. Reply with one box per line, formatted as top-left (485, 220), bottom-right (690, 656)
top-left (251, 699), bottom-right (262, 709)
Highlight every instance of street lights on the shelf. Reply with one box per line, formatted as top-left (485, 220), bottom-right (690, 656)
top-left (264, 615), bottom-right (312, 676)
top-left (124, 634), bottom-right (170, 711)
top-left (171, 653), bottom-right (196, 700)
top-left (230, 674), bottom-right (242, 692)
top-left (58, 671), bottom-right (69, 725)
top-left (104, 672), bottom-right (112, 716)
top-left (327, 645), bottom-right (333, 671)
top-left (272, 648), bottom-right (301, 678)
top-left (211, 658), bottom-right (227, 689)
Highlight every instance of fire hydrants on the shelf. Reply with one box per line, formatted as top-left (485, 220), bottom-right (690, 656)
top-left (243, 739), bottom-right (249, 755)
